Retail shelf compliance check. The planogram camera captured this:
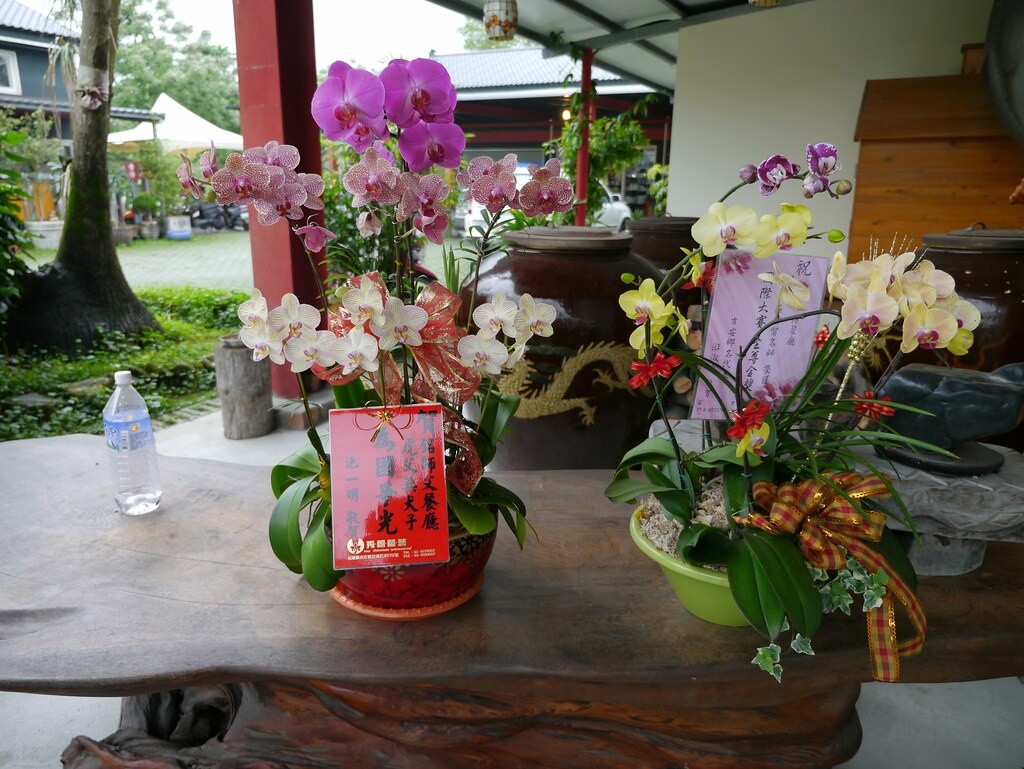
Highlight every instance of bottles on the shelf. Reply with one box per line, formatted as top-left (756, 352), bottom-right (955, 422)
top-left (101, 371), bottom-right (163, 515)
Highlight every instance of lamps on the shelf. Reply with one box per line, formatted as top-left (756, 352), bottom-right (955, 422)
top-left (484, 0), bottom-right (519, 41)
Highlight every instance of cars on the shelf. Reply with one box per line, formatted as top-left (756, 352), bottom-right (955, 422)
top-left (451, 189), bottom-right (464, 236)
top-left (464, 167), bottom-right (633, 237)
top-left (124, 202), bottom-right (137, 224)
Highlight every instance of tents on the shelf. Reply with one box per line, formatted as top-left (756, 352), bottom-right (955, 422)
top-left (108, 93), bottom-right (244, 150)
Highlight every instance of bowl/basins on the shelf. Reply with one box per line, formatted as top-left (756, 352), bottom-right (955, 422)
top-left (220, 333), bottom-right (245, 348)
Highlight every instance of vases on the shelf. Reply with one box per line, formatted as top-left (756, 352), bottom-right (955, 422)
top-left (630, 505), bottom-right (751, 627)
top-left (329, 504), bottom-right (500, 620)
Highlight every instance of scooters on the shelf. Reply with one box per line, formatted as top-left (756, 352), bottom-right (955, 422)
top-left (182, 198), bottom-right (250, 231)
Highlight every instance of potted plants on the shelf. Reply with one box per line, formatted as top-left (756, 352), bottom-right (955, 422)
top-left (133, 192), bottom-right (160, 240)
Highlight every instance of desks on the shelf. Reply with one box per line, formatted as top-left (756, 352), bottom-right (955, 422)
top-left (845, 137), bottom-right (1024, 263)
top-left (1, 433), bottom-right (1024, 769)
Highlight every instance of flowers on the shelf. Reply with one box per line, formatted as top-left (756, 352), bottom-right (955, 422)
top-left (604, 145), bottom-right (981, 682)
top-left (177, 58), bottom-right (575, 592)
